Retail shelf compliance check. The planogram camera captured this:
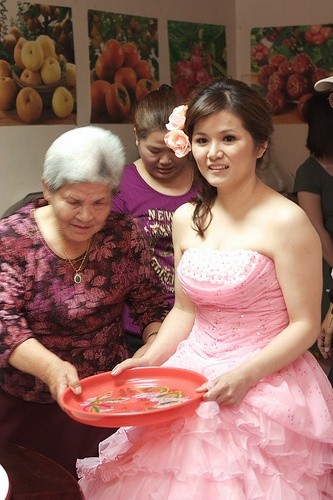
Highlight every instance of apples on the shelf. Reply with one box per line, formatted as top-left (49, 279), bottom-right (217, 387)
top-left (0, 2), bottom-right (77, 123)
top-left (250, 24), bottom-right (333, 120)
top-left (87, 9), bottom-right (158, 122)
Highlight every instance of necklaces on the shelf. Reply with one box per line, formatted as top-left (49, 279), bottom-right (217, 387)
top-left (54, 228), bottom-right (92, 284)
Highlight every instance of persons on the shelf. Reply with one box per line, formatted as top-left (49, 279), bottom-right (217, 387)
top-left (315, 75), bottom-right (333, 386)
top-left (293, 90), bottom-right (332, 351)
top-left (75, 77), bottom-right (333, 500)
top-left (108, 83), bottom-right (203, 359)
top-left (1, 126), bottom-right (169, 481)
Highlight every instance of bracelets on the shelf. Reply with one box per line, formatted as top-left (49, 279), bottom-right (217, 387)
top-left (145, 332), bottom-right (158, 339)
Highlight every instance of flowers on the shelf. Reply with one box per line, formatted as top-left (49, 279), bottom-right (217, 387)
top-left (164, 105), bottom-right (191, 158)
